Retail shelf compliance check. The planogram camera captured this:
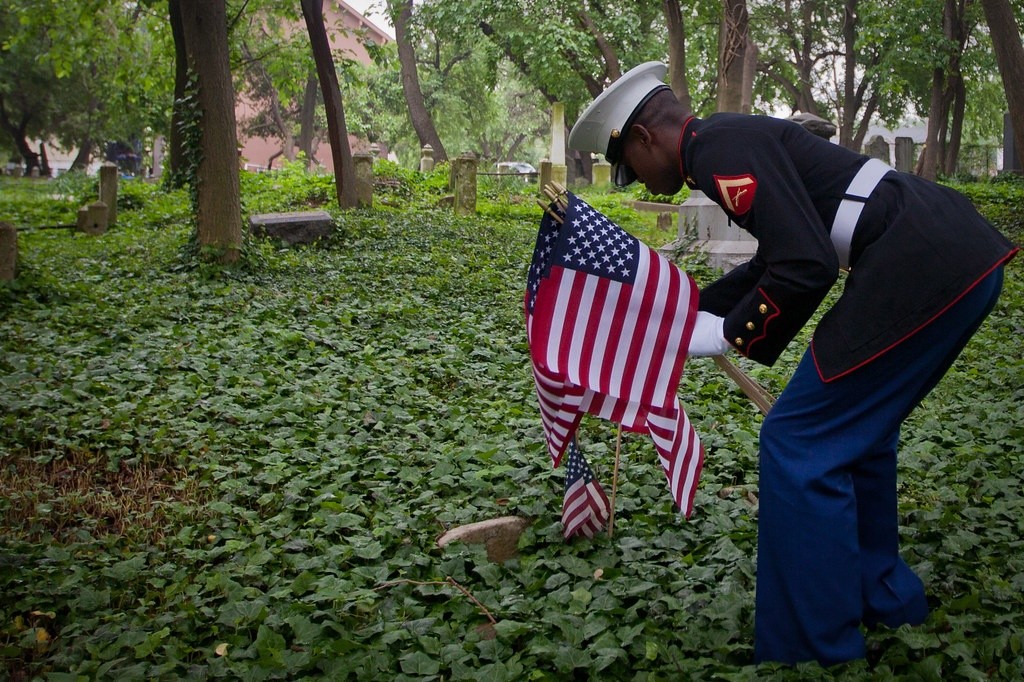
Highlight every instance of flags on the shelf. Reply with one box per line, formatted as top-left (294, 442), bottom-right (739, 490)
top-left (523, 190), bottom-right (705, 540)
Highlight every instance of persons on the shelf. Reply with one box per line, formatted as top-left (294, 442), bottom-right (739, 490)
top-left (568, 61), bottom-right (1020, 670)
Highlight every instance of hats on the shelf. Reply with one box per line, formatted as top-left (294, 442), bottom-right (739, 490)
top-left (564, 60), bottom-right (673, 189)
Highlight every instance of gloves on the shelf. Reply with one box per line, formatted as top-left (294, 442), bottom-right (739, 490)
top-left (686, 311), bottom-right (731, 356)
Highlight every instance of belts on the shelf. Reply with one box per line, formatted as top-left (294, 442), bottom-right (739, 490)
top-left (828, 157), bottom-right (897, 273)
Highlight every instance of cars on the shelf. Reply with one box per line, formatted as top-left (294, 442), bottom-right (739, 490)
top-left (491, 162), bottom-right (538, 184)
